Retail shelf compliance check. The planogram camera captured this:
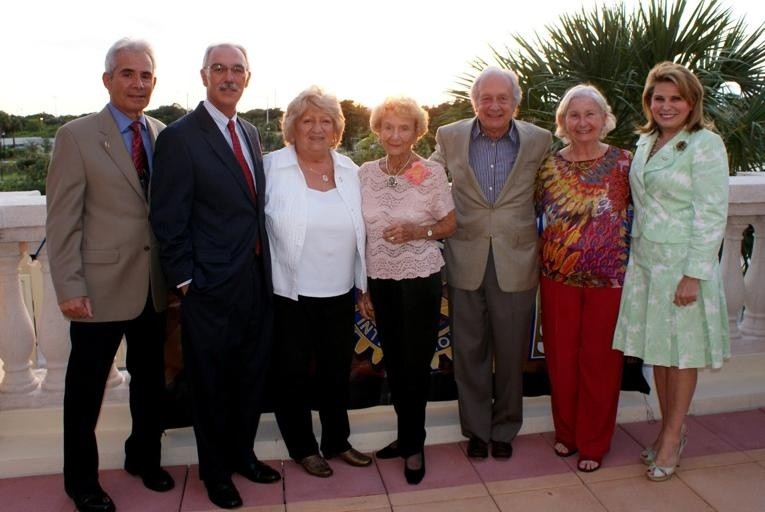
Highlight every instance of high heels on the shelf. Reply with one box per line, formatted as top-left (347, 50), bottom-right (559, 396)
top-left (403, 445), bottom-right (426, 486)
top-left (639, 421), bottom-right (688, 466)
top-left (645, 434), bottom-right (688, 481)
top-left (376, 429), bottom-right (427, 459)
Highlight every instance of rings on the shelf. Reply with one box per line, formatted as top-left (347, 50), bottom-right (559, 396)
top-left (389, 236), bottom-right (396, 242)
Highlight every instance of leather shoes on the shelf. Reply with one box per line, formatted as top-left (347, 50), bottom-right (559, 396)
top-left (236, 457), bottom-right (282, 485)
top-left (122, 456), bottom-right (175, 492)
top-left (338, 447), bottom-right (372, 467)
top-left (63, 482), bottom-right (116, 512)
top-left (299, 453), bottom-right (334, 478)
top-left (467, 435), bottom-right (489, 460)
top-left (203, 476), bottom-right (242, 510)
top-left (490, 437), bottom-right (514, 460)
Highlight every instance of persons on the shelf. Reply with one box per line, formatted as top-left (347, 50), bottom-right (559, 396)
top-left (263, 89), bottom-right (372, 477)
top-left (611, 61), bottom-right (729, 480)
top-left (535, 84), bottom-right (635, 471)
top-left (354, 98), bottom-right (456, 484)
top-left (147, 43), bottom-right (281, 508)
top-left (48, 38), bottom-right (174, 511)
top-left (430, 68), bottom-right (553, 461)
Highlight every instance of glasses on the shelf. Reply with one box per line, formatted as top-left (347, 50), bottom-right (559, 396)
top-left (204, 63), bottom-right (248, 74)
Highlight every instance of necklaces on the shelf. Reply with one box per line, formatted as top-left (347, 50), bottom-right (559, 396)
top-left (569, 144), bottom-right (601, 170)
top-left (650, 133), bottom-right (676, 160)
top-left (298, 160), bottom-right (334, 183)
top-left (386, 154), bottom-right (414, 189)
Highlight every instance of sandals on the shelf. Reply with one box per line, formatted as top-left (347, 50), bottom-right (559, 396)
top-left (577, 455), bottom-right (603, 473)
top-left (554, 440), bottom-right (578, 458)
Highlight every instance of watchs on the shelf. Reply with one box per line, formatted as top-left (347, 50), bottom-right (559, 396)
top-left (427, 226), bottom-right (432, 242)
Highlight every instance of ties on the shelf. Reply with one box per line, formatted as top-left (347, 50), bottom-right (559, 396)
top-left (127, 122), bottom-right (151, 204)
top-left (226, 119), bottom-right (258, 205)
